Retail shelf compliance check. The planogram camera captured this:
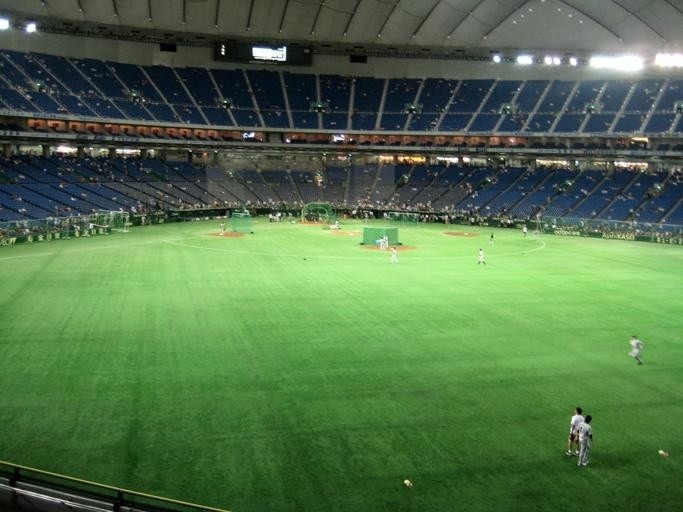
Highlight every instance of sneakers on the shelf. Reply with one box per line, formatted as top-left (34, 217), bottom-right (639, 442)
top-left (565, 450), bottom-right (590, 468)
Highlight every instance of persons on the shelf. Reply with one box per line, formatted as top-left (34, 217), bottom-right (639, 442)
top-left (563, 407), bottom-right (584, 457)
top-left (476, 247), bottom-right (485, 265)
top-left (574, 414), bottom-right (593, 468)
top-left (1, 49), bottom-right (683, 249)
top-left (626, 335), bottom-right (645, 366)
top-left (389, 244), bottom-right (399, 264)
